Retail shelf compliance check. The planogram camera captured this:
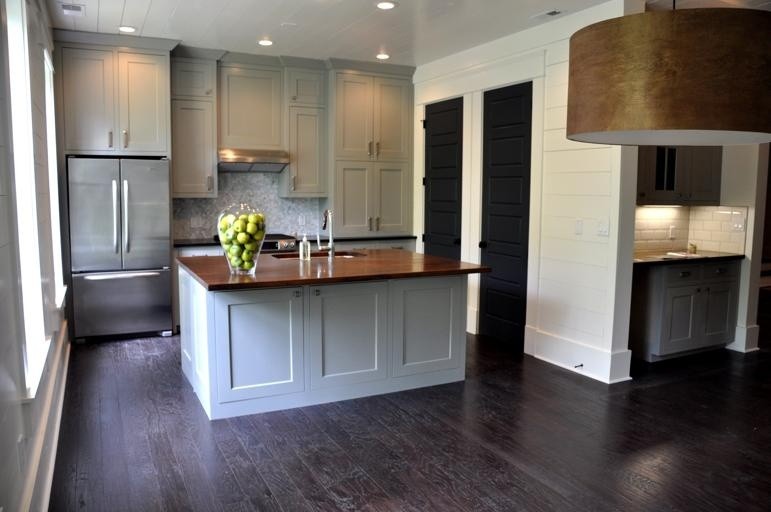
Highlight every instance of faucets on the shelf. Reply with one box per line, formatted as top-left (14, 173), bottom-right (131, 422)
top-left (317, 257), bottom-right (335, 278)
top-left (315, 208), bottom-right (336, 256)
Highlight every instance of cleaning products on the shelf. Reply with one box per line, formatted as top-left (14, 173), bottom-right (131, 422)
top-left (300, 261), bottom-right (311, 279)
top-left (299, 233), bottom-right (311, 261)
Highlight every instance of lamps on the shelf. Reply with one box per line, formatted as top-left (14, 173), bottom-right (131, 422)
top-left (560, 1), bottom-right (768, 149)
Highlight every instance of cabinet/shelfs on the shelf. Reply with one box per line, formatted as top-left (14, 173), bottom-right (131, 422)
top-left (317, 164), bottom-right (415, 239)
top-left (54, 43), bottom-right (173, 160)
top-left (388, 274), bottom-right (466, 394)
top-left (278, 71), bottom-right (329, 198)
top-left (333, 72), bottom-right (414, 162)
top-left (630, 259), bottom-right (741, 363)
top-left (638, 145), bottom-right (723, 205)
top-left (217, 64), bottom-right (285, 151)
top-left (177, 267), bottom-right (386, 422)
top-left (170, 55), bottom-right (219, 201)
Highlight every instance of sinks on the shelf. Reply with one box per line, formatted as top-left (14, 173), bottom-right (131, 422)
top-left (272, 251), bottom-right (368, 259)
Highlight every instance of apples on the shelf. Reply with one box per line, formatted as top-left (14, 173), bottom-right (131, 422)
top-left (218, 212), bottom-right (265, 273)
